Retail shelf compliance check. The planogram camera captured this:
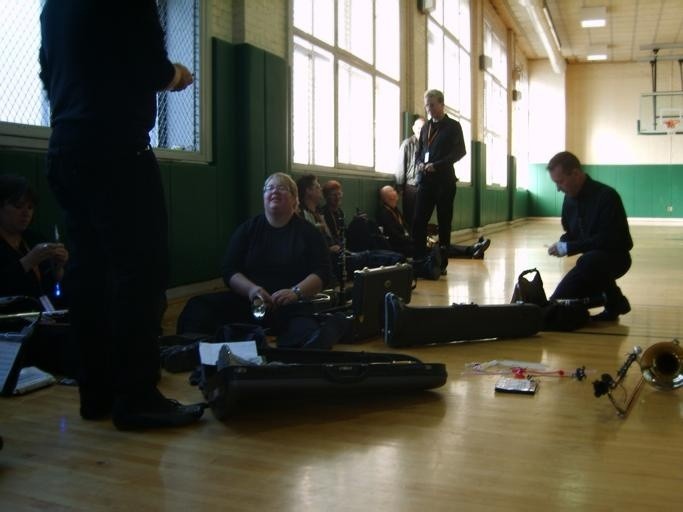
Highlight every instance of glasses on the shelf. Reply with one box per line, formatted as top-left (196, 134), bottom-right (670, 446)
top-left (264, 185), bottom-right (293, 193)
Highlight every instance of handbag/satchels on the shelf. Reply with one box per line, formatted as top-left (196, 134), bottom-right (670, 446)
top-left (511, 269), bottom-right (548, 305)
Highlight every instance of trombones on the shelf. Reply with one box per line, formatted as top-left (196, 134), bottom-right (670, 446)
top-left (608, 339), bottom-right (683, 417)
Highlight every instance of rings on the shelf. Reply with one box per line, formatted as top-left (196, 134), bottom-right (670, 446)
top-left (43, 244), bottom-right (49, 249)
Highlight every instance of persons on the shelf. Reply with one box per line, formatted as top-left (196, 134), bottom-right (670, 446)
top-left (394, 89), bottom-right (467, 276)
top-left (178, 172), bottom-right (491, 375)
top-left (39, 0), bottom-right (205, 429)
top-left (547, 151), bottom-right (633, 322)
top-left (0, 175), bottom-right (77, 381)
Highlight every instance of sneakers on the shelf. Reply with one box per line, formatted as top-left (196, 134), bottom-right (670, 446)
top-left (304, 312), bottom-right (346, 349)
top-left (81, 396), bottom-right (203, 431)
top-left (164, 338), bottom-right (212, 369)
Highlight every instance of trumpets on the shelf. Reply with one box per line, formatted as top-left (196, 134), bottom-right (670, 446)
top-left (251, 286), bottom-right (341, 321)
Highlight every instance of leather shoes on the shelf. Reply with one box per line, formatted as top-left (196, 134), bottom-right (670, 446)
top-left (472, 236), bottom-right (489, 259)
top-left (591, 297), bottom-right (630, 321)
top-left (425, 246), bottom-right (449, 279)
